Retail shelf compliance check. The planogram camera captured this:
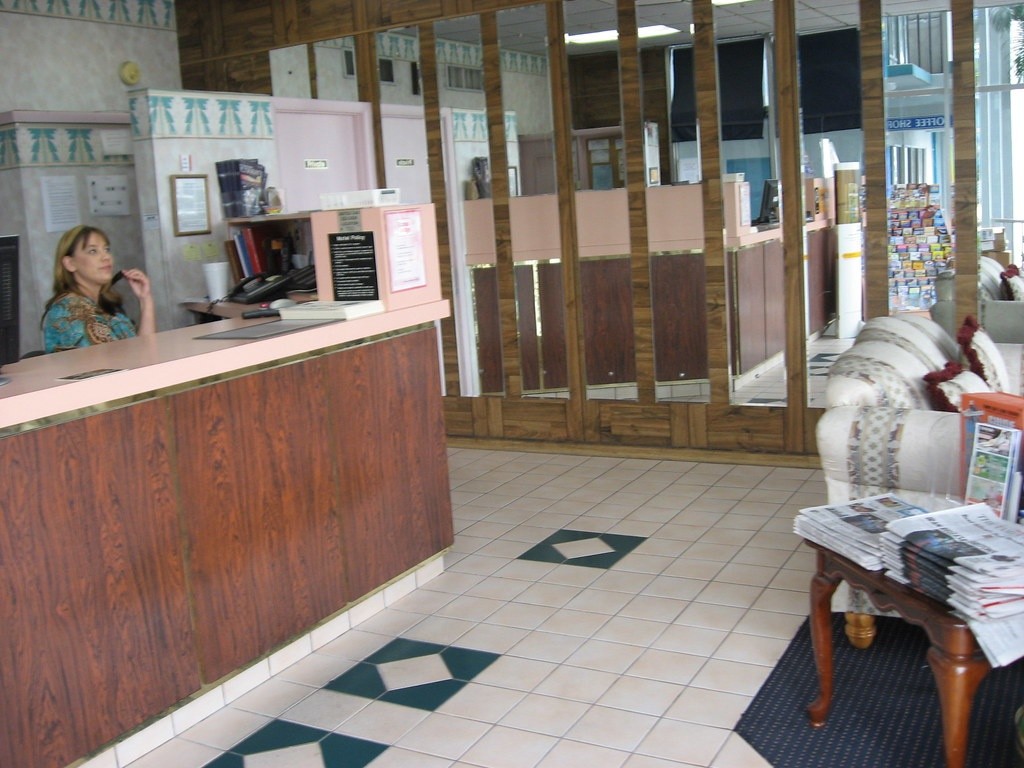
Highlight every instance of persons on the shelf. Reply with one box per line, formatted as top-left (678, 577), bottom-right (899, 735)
top-left (44, 226), bottom-right (155, 354)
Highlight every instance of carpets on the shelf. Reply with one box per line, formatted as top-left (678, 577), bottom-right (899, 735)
top-left (737, 606), bottom-right (1024, 768)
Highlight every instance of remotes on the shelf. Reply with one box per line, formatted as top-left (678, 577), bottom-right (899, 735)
top-left (240, 309), bottom-right (280, 320)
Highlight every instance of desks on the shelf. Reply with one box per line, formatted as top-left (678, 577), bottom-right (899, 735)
top-left (800, 538), bottom-right (992, 768)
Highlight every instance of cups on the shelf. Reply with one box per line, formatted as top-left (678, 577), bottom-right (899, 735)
top-left (202, 261), bottom-right (229, 302)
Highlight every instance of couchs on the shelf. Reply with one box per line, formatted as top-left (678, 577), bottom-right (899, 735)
top-left (818, 308), bottom-right (1024, 506)
top-left (932, 254), bottom-right (1024, 341)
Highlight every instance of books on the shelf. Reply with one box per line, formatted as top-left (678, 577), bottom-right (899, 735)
top-left (859, 175), bottom-right (956, 311)
top-left (279, 300), bottom-right (383, 320)
top-left (216, 159), bottom-right (268, 216)
top-left (224, 225), bottom-right (291, 281)
top-left (964, 422), bottom-right (1022, 520)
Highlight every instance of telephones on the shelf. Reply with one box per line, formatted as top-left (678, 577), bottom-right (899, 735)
top-left (229, 273), bottom-right (286, 305)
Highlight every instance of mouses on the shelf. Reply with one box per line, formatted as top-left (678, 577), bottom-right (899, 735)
top-left (270, 298), bottom-right (298, 310)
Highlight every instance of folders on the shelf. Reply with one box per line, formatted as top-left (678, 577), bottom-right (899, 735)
top-left (225, 227), bottom-right (278, 279)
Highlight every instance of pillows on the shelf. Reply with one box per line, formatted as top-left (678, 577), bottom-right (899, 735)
top-left (923, 361), bottom-right (996, 412)
top-left (957, 317), bottom-right (1006, 392)
top-left (1000, 262), bottom-right (1023, 300)
